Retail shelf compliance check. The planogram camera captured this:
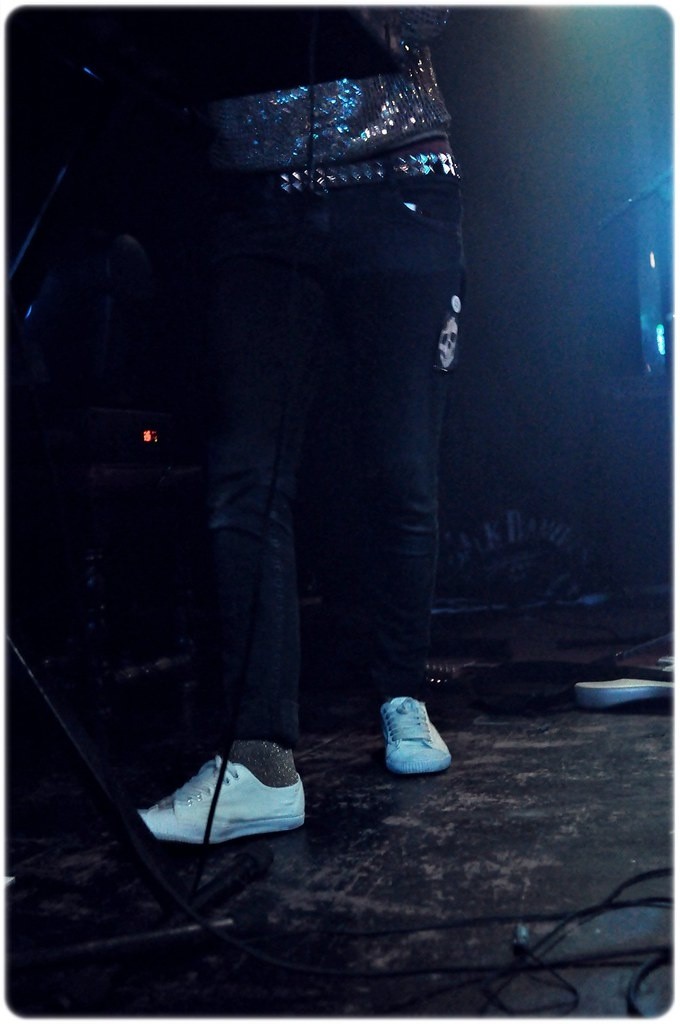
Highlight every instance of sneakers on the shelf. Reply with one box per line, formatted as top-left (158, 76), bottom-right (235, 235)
top-left (379, 695), bottom-right (451, 772)
top-left (137, 755), bottom-right (306, 843)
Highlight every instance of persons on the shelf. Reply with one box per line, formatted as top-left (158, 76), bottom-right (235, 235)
top-left (136, 7), bottom-right (464, 844)
top-left (21, 200), bottom-right (188, 814)
